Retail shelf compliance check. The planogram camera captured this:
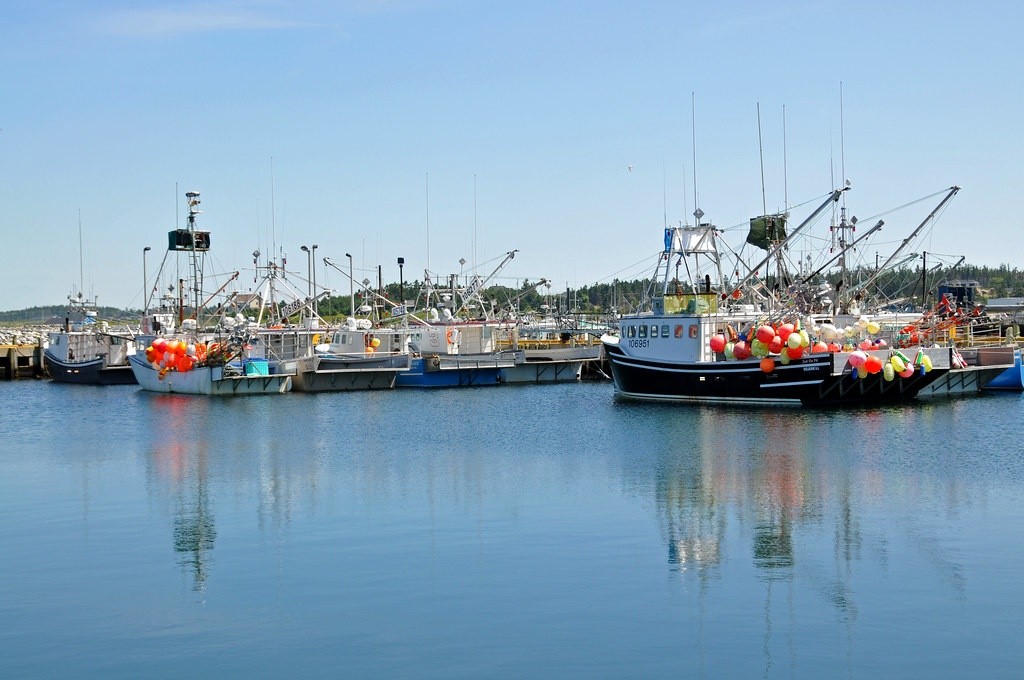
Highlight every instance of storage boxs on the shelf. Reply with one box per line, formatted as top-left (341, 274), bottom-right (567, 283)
top-left (245, 361), bottom-right (269, 375)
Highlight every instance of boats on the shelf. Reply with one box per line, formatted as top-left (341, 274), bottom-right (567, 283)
top-left (601, 178), bottom-right (953, 408)
top-left (723, 179), bottom-right (1024, 394)
top-left (2, 190), bottom-right (655, 394)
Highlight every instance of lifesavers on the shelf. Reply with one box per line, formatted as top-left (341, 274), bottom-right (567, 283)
top-left (447, 330), bottom-right (459, 344)
top-left (197, 344), bottom-right (206, 354)
